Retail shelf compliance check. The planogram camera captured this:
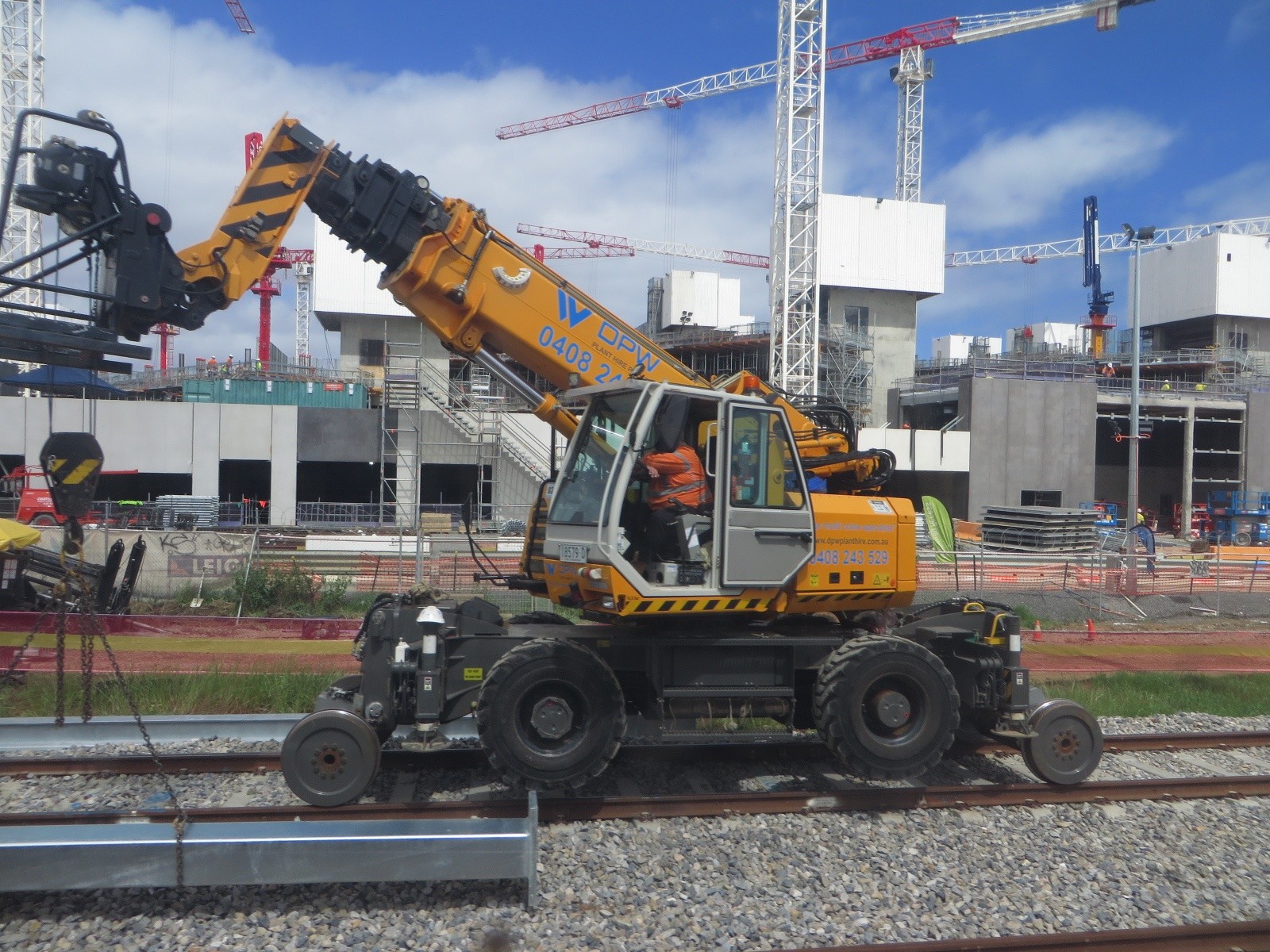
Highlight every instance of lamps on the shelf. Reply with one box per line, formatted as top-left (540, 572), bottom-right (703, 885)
top-left (1166, 244), bottom-right (1173, 250)
top-left (877, 198), bottom-right (883, 204)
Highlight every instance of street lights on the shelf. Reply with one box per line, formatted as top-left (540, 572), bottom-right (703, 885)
top-left (1120, 221), bottom-right (1156, 597)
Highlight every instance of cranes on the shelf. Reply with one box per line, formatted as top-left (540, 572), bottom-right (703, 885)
top-left (946, 196), bottom-right (1270, 373)
top-left (492, 0), bottom-right (1160, 203)
top-left (766, 1), bottom-right (829, 406)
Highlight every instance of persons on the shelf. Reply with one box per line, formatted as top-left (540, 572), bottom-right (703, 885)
top-left (1137, 508), bottom-right (1144, 525)
top-left (221, 366), bottom-right (229, 374)
top-left (903, 422), bottom-right (909, 428)
top-left (1161, 379), bottom-right (1169, 390)
top-left (226, 354), bottom-right (233, 369)
top-left (207, 356), bottom-right (217, 376)
top-left (1101, 363), bottom-right (1117, 386)
top-left (626, 419), bottom-right (714, 562)
top-left (256, 358), bottom-right (261, 370)
top-left (1196, 382), bottom-right (1206, 391)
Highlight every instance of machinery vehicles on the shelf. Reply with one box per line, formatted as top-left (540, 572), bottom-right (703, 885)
top-left (1, 102), bottom-right (1108, 812)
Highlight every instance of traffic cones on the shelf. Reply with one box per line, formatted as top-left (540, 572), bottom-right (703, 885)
top-left (1082, 618), bottom-right (1100, 641)
top-left (1028, 620), bottom-right (1046, 642)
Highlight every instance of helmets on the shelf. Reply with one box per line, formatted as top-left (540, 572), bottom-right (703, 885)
top-left (1164, 380), bottom-right (1169, 384)
top-left (212, 356), bottom-right (215, 359)
top-left (903, 423), bottom-right (910, 429)
top-left (229, 354), bottom-right (233, 357)
top-left (1107, 363), bottom-right (1112, 368)
top-left (1137, 508), bottom-right (1142, 512)
top-left (256, 358), bottom-right (261, 360)
